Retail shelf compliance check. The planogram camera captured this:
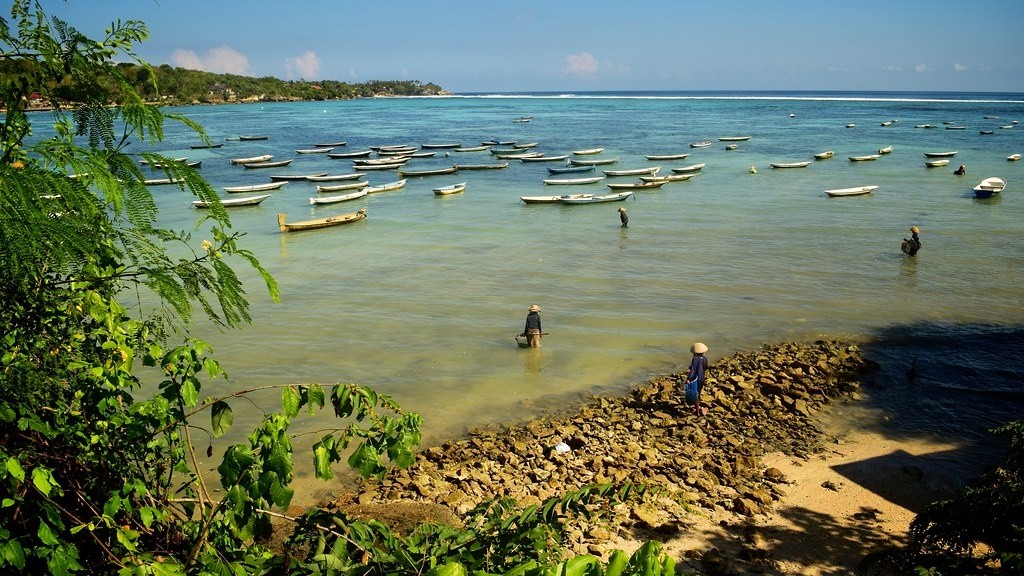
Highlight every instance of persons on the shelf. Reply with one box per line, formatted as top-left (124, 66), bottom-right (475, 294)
top-left (524, 305), bottom-right (542, 347)
top-left (685, 343), bottom-right (708, 417)
top-left (903, 227), bottom-right (921, 257)
top-left (617, 207), bottom-right (628, 228)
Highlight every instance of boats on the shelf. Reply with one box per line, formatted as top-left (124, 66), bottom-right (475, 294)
top-left (225, 135), bottom-right (269, 141)
top-left (848, 154), bottom-right (882, 162)
top-left (309, 185), bottom-right (370, 206)
top-left (222, 180), bottom-right (290, 194)
top-left (973, 176), bottom-right (1008, 200)
top-left (769, 161), bottom-right (813, 169)
top-left (192, 193), bottom-right (273, 210)
top-left (1006, 153), bottom-right (1022, 161)
top-left (189, 143), bottom-right (225, 151)
top-left (243, 159), bottom-right (294, 169)
top-left (229, 154), bottom-right (274, 165)
top-left (305, 172), bottom-right (368, 182)
top-left (270, 172), bottom-right (329, 182)
top-left (138, 156), bottom-right (190, 167)
top-left (154, 159), bottom-right (203, 169)
top-left (718, 135), bottom-right (752, 141)
top-left (813, 150), bottom-right (836, 160)
top-left (115, 177), bottom-right (185, 186)
top-left (689, 139), bottom-right (713, 148)
top-left (824, 185), bottom-right (879, 197)
top-left (294, 138), bottom-right (707, 206)
top-left (845, 115), bottom-right (1019, 168)
top-left (284, 208), bottom-right (369, 232)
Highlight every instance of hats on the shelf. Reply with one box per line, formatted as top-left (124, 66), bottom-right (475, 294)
top-left (910, 226), bottom-right (919, 234)
top-left (690, 343), bottom-right (709, 353)
top-left (617, 207), bottom-right (626, 212)
top-left (529, 305), bottom-right (540, 311)
top-left (960, 164), bottom-right (965, 168)
